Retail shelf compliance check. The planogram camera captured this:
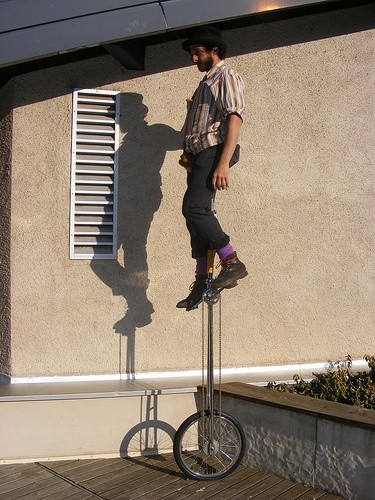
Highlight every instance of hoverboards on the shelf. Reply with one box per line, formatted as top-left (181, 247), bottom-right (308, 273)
top-left (174, 166), bottom-right (245, 484)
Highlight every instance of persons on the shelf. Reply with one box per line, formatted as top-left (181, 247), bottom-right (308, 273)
top-left (173, 24), bottom-right (249, 311)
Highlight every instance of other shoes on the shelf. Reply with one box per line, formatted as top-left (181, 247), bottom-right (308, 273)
top-left (214, 252), bottom-right (247, 286)
top-left (176, 275), bottom-right (213, 308)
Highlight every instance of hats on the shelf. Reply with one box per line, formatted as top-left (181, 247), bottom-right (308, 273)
top-left (182, 27), bottom-right (229, 53)
